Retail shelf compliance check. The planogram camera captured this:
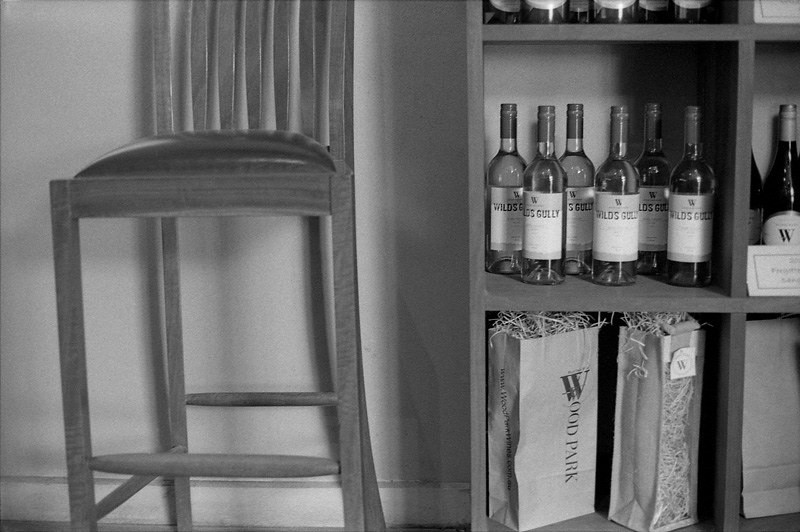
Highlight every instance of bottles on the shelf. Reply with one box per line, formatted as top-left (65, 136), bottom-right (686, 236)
top-left (558, 103), bottom-right (596, 274)
top-left (749, 146), bottom-right (763, 246)
top-left (521, 105), bottom-right (568, 286)
top-left (664, 106), bottom-right (716, 287)
top-left (482, 0), bottom-right (710, 25)
top-left (760, 104), bottom-right (800, 245)
top-left (591, 105), bottom-right (640, 286)
top-left (634, 102), bottom-right (674, 274)
top-left (485, 103), bottom-right (528, 274)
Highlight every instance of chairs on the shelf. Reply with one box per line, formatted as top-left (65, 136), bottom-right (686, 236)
top-left (50, 0), bottom-right (385, 532)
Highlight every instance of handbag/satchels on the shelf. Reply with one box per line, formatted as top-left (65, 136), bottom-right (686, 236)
top-left (742, 315), bottom-right (800, 518)
top-left (487, 326), bottom-right (598, 531)
top-left (608, 314), bottom-right (706, 532)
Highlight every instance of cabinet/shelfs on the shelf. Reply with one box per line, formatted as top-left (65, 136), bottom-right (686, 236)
top-left (466, 0), bottom-right (800, 532)
